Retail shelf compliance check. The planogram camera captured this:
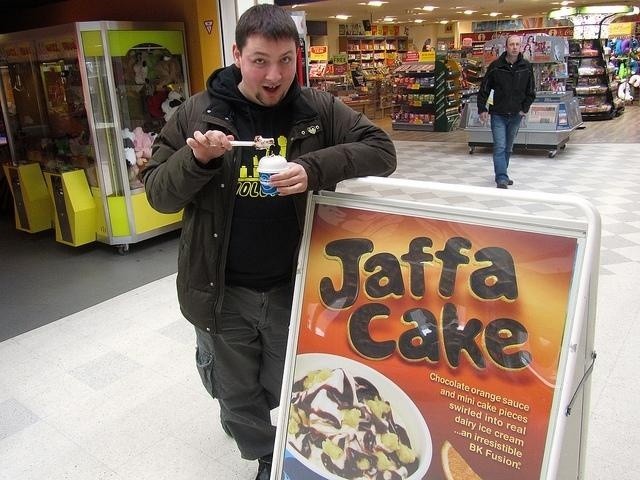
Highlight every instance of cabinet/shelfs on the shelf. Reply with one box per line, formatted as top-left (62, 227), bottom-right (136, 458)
top-left (308, 34), bottom-right (466, 131)
top-left (567, 39), bottom-right (627, 120)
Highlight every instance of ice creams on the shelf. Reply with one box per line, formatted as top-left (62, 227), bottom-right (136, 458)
top-left (258, 153), bottom-right (289, 195)
top-left (253, 135), bottom-right (274, 150)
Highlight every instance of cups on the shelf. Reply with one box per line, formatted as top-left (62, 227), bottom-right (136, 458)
top-left (255, 155), bottom-right (288, 196)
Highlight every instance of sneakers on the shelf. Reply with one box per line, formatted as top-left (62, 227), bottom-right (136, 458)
top-left (508, 180), bottom-right (513, 185)
top-left (255, 453), bottom-right (272, 479)
top-left (497, 184), bottom-right (507, 189)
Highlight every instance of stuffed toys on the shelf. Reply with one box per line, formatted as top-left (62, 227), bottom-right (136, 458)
top-left (608, 36), bottom-right (640, 102)
top-left (22, 43), bottom-right (184, 192)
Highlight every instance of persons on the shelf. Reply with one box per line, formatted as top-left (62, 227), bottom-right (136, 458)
top-left (522, 44), bottom-right (532, 62)
top-left (527, 36), bottom-right (536, 45)
top-left (478, 36), bottom-right (535, 189)
top-left (144, 4), bottom-right (400, 479)
